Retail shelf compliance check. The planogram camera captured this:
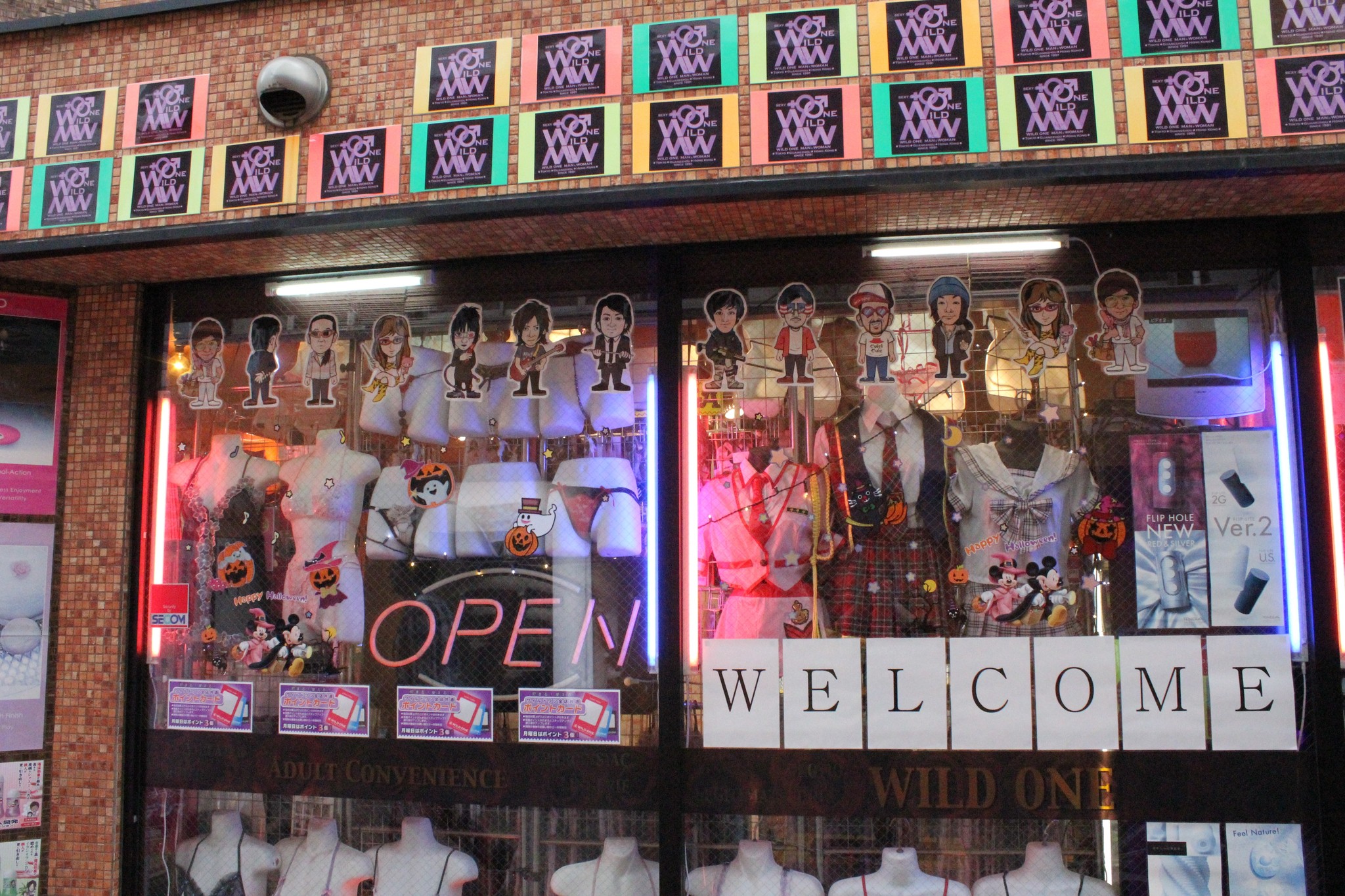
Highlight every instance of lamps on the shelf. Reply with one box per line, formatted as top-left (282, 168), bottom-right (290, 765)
top-left (263, 268), bottom-right (433, 298)
top-left (862, 233), bottom-right (1067, 259)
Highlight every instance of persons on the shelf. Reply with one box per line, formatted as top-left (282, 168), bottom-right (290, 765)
top-left (273, 816), bottom-right (373, 896)
top-left (170, 432), bottom-right (280, 637)
top-left (276, 427), bottom-right (383, 648)
top-left (454, 461), bottom-right (546, 559)
top-left (814, 384), bottom-right (961, 638)
top-left (359, 344), bottom-right (452, 446)
top-left (737, 315), bottom-right (841, 420)
top-left (550, 835), bottom-right (660, 896)
top-left (365, 465), bottom-right (457, 562)
top-left (446, 342), bottom-right (541, 438)
top-left (544, 457), bottom-right (642, 558)
top-left (949, 419), bottom-right (1102, 637)
top-left (539, 332), bottom-right (635, 440)
top-left (685, 839), bottom-right (826, 896)
top-left (698, 447), bottom-right (834, 639)
top-left (984, 304), bottom-right (1086, 421)
top-left (364, 818), bottom-right (479, 896)
top-left (829, 847), bottom-right (972, 896)
top-left (973, 840), bottom-right (1117, 896)
top-left (174, 808), bottom-right (281, 896)
top-left (847, 313), bottom-right (965, 421)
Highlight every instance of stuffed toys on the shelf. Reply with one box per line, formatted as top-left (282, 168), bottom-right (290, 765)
top-left (239, 607), bottom-right (275, 673)
top-left (1025, 555), bottom-right (1076, 627)
top-left (273, 614), bottom-right (307, 678)
top-left (980, 553), bottom-right (1028, 627)
